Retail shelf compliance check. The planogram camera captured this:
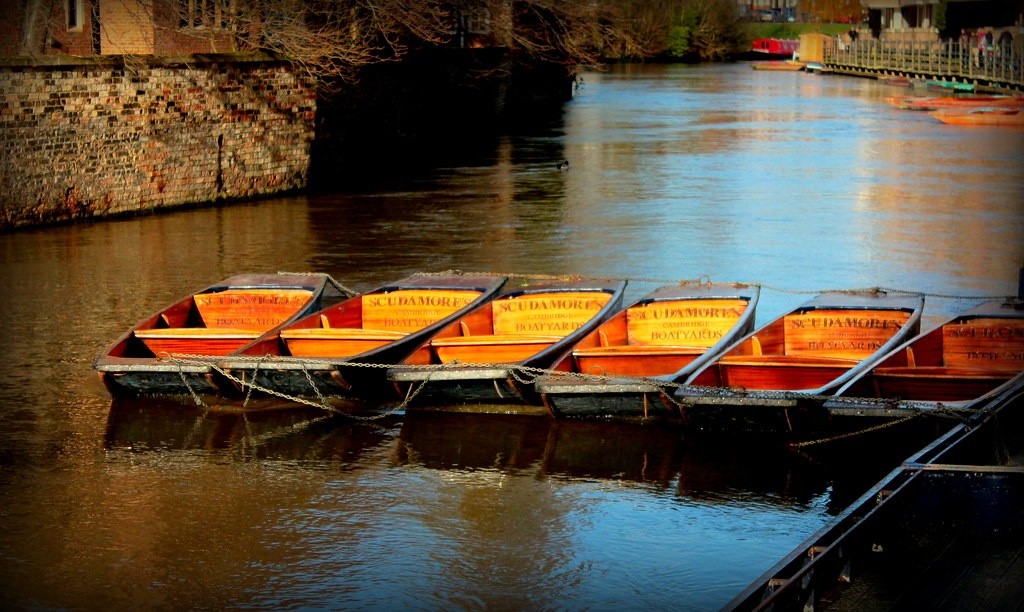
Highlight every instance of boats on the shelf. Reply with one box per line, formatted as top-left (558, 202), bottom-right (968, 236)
top-left (215, 272), bottom-right (510, 406)
top-left (386, 276), bottom-right (629, 415)
top-left (896, 95), bottom-right (1024, 111)
top-left (822, 267), bottom-right (1024, 447)
top-left (928, 105), bottom-right (1024, 126)
top-left (673, 286), bottom-right (925, 436)
top-left (91, 271), bottom-right (330, 402)
top-left (537, 279), bottom-right (761, 424)
